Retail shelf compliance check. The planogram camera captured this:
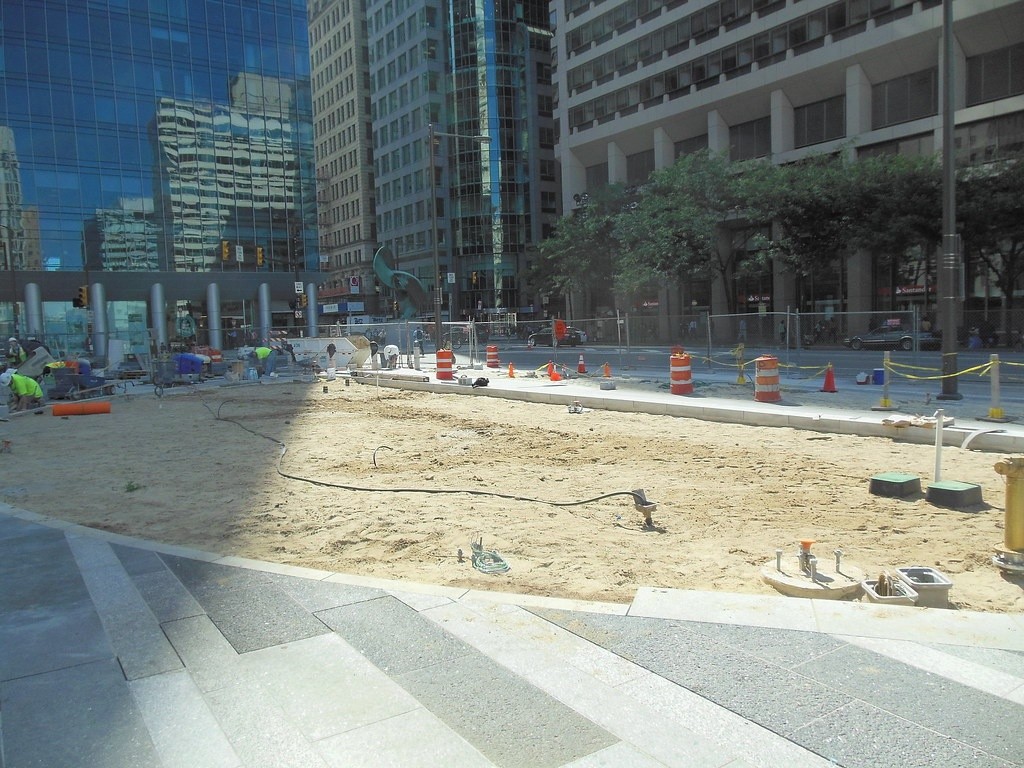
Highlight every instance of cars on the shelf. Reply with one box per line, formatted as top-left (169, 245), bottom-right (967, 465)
top-left (442, 325), bottom-right (490, 346)
top-left (528, 328), bottom-right (581, 348)
top-left (841, 324), bottom-right (942, 351)
top-left (563, 326), bottom-right (588, 345)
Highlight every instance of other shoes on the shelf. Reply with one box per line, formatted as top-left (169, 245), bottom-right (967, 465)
top-left (38, 399), bottom-right (46, 407)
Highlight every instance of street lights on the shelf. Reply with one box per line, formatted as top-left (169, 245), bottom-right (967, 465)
top-left (424, 120), bottom-right (493, 349)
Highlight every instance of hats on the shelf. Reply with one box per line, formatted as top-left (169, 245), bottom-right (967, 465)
top-left (248, 351), bottom-right (253, 358)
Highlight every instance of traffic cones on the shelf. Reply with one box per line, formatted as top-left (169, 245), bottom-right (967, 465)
top-left (574, 355), bottom-right (587, 374)
top-left (818, 361), bottom-right (839, 394)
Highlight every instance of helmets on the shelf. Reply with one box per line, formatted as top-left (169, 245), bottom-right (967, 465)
top-left (9, 338), bottom-right (16, 342)
top-left (0, 373), bottom-right (11, 386)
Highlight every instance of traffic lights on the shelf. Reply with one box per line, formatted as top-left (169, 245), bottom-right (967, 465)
top-left (301, 294), bottom-right (309, 308)
top-left (221, 240), bottom-right (231, 261)
top-left (472, 270), bottom-right (479, 285)
top-left (78, 286), bottom-right (87, 307)
top-left (255, 245), bottom-right (265, 267)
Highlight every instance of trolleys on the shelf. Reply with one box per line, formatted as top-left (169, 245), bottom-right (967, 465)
top-left (48, 373), bottom-right (136, 400)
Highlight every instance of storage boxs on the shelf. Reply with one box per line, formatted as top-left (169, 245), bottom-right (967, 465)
top-left (861, 567), bottom-right (951, 608)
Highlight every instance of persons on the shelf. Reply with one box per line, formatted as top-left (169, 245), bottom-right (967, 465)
top-left (779, 319), bottom-right (785, 345)
top-left (0, 337), bottom-right (66, 412)
top-left (920, 317), bottom-right (931, 332)
top-left (384, 345), bottom-right (399, 368)
top-left (814, 317), bottom-right (837, 343)
top-left (413, 327), bottom-right (424, 357)
top-left (736, 316), bottom-right (747, 342)
top-left (248, 347), bottom-right (272, 378)
top-left (226, 320), bottom-right (240, 349)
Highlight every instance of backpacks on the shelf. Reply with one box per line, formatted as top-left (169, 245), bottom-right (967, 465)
top-left (416, 329), bottom-right (422, 339)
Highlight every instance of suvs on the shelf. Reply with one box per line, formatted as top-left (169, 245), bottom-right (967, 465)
top-left (441, 325), bottom-right (488, 344)
